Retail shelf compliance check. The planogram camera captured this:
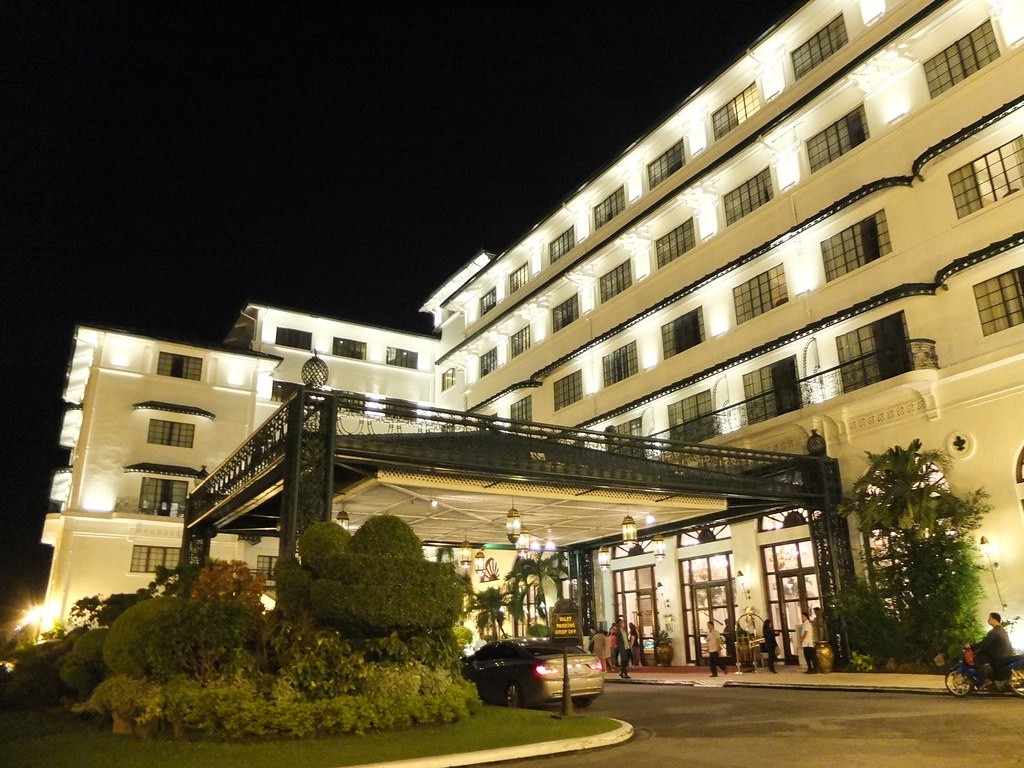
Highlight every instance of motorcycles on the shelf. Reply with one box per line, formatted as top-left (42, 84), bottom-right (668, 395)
top-left (945, 644), bottom-right (1024, 699)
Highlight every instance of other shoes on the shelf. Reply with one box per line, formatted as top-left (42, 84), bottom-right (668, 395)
top-left (710, 674), bottom-right (717, 677)
top-left (625, 676), bottom-right (631, 678)
top-left (619, 673), bottom-right (624, 678)
top-left (770, 667), bottom-right (777, 673)
top-left (972, 677), bottom-right (979, 687)
top-left (804, 670), bottom-right (817, 674)
top-left (724, 668), bottom-right (727, 674)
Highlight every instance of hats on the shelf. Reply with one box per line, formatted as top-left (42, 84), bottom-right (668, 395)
top-left (615, 619), bottom-right (625, 626)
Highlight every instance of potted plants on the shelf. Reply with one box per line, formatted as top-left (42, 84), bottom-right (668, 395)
top-left (651, 630), bottom-right (673, 666)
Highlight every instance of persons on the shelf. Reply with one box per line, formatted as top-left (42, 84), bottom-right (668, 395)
top-left (762, 619), bottom-right (780, 674)
top-left (963, 612), bottom-right (1014, 690)
top-left (799, 607), bottom-right (825, 674)
top-left (588, 619), bottom-right (736, 678)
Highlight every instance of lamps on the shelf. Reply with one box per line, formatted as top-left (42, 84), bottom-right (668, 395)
top-left (506, 509), bottom-right (520, 544)
top-left (622, 516), bottom-right (638, 545)
top-left (597, 545), bottom-right (610, 568)
top-left (336, 512), bottom-right (349, 530)
top-left (651, 534), bottom-right (666, 562)
top-left (474, 551), bottom-right (485, 576)
top-left (459, 540), bottom-right (472, 566)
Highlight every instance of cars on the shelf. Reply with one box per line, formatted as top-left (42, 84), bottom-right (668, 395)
top-left (459, 635), bottom-right (605, 707)
